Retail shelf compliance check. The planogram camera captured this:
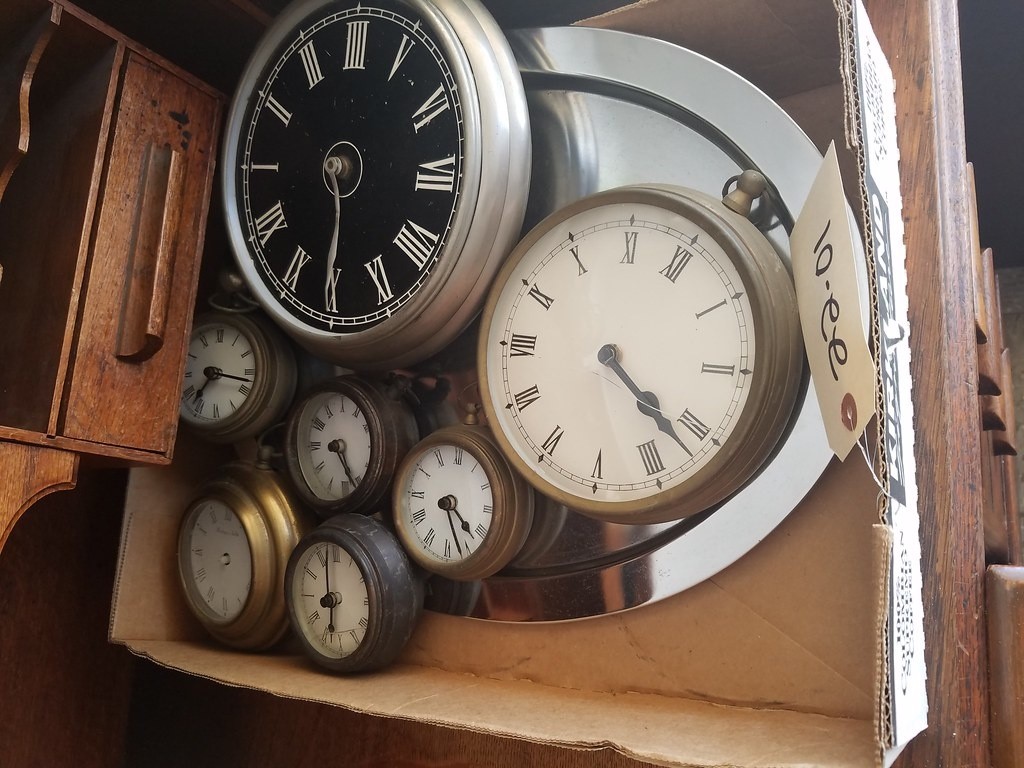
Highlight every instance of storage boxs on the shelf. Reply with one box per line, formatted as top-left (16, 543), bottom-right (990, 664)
top-left (104, 0), bottom-right (929, 768)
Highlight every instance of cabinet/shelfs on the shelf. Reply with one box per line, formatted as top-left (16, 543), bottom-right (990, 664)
top-left (0, 0), bottom-right (227, 552)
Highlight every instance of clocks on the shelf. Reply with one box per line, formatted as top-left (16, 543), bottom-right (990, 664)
top-left (476, 170), bottom-right (808, 525)
top-left (282, 375), bottom-right (421, 516)
top-left (178, 303), bottom-right (298, 445)
top-left (391, 423), bottom-right (533, 583)
top-left (218, 0), bottom-right (534, 372)
top-left (283, 512), bottom-right (425, 674)
top-left (175, 459), bottom-right (316, 653)
